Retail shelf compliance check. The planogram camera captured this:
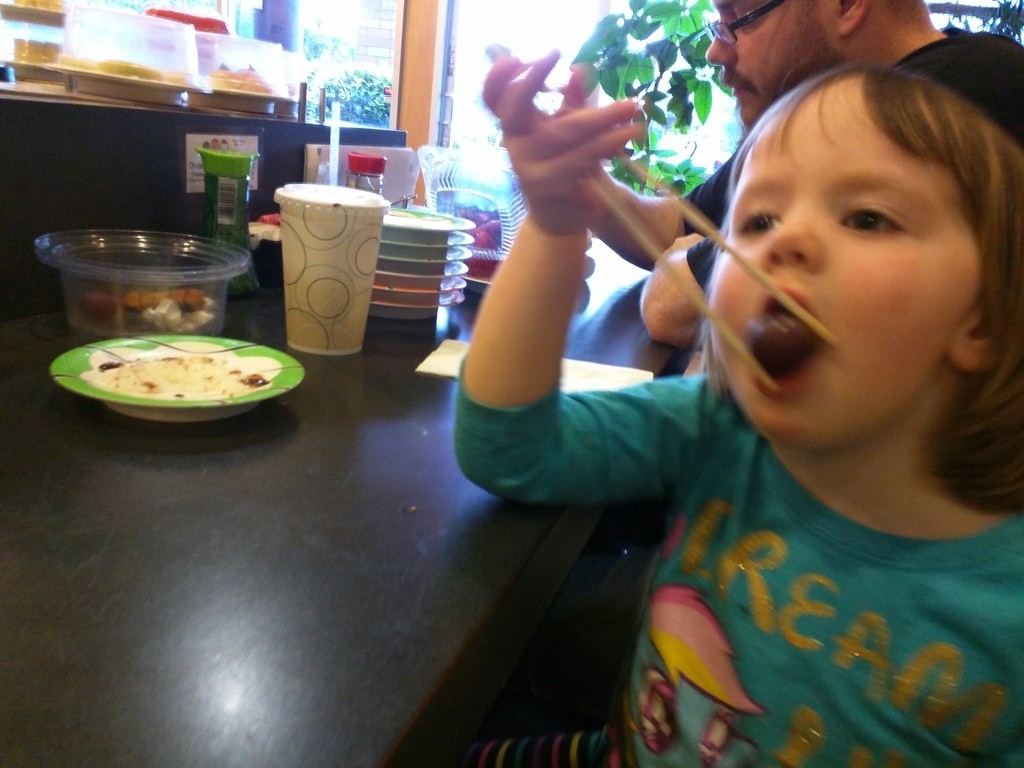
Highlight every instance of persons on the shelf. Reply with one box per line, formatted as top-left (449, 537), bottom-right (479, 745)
top-left (452, 50), bottom-right (1024, 768)
top-left (587, 0), bottom-right (1024, 359)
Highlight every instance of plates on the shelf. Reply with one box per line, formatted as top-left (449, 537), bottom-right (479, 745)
top-left (368, 209), bottom-right (477, 319)
top-left (50, 335), bottom-right (304, 422)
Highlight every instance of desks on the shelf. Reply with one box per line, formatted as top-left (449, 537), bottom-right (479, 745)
top-left (0, 237), bottom-right (675, 768)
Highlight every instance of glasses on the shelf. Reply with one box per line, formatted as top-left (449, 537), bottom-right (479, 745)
top-left (702, 0), bottom-right (786, 45)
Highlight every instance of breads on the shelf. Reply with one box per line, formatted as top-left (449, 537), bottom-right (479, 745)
top-left (12, 0), bottom-right (275, 96)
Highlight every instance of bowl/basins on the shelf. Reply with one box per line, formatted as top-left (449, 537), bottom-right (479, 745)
top-left (35, 231), bottom-right (252, 335)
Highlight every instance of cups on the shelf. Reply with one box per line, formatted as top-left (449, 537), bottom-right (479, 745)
top-left (275, 183), bottom-right (391, 355)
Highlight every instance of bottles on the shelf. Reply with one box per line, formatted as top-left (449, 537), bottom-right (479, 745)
top-left (346, 154), bottom-right (388, 195)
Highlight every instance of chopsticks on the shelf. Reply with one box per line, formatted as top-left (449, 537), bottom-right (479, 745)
top-left (585, 156), bottom-right (842, 395)
